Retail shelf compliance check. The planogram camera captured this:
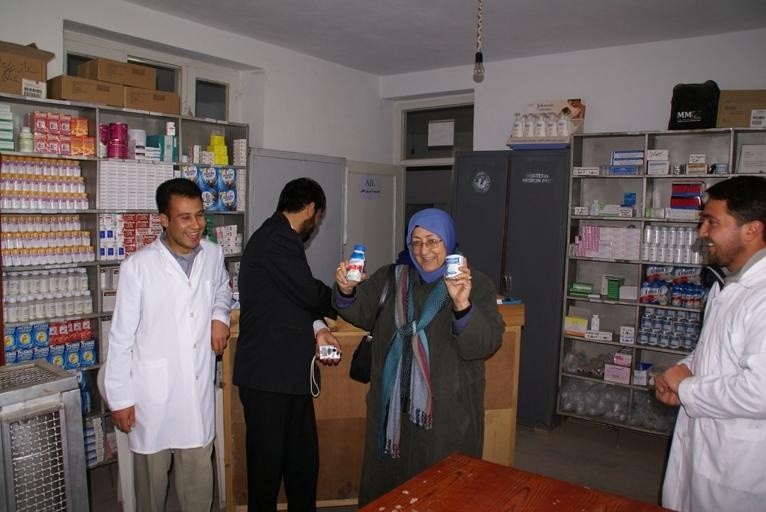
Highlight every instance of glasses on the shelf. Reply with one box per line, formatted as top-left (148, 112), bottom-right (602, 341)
top-left (407, 239), bottom-right (442, 248)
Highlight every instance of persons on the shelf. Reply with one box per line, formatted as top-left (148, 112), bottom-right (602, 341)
top-left (331, 208), bottom-right (505, 508)
top-left (103, 178), bottom-right (234, 512)
top-left (654, 175), bottom-right (766, 511)
top-left (229, 178), bottom-right (344, 512)
top-left (560, 107), bottom-right (573, 119)
top-left (567, 99), bottom-right (586, 120)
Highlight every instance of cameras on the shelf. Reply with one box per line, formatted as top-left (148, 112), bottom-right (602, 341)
top-left (319, 345), bottom-right (340, 359)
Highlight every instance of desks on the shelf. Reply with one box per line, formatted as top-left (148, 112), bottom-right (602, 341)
top-left (360, 451), bottom-right (676, 510)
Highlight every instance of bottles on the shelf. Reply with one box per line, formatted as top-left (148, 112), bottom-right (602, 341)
top-left (344, 241), bottom-right (368, 284)
top-left (0, 154), bottom-right (93, 210)
top-left (632, 388), bottom-right (679, 435)
top-left (0, 213), bottom-right (97, 267)
top-left (561, 348), bottom-right (615, 377)
top-left (559, 375), bottom-right (632, 426)
top-left (512, 111), bottom-right (575, 145)
top-left (590, 314), bottom-right (601, 332)
top-left (1, 264), bottom-right (95, 325)
top-left (17, 125), bottom-right (36, 153)
top-left (640, 224), bottom-right (710, 264)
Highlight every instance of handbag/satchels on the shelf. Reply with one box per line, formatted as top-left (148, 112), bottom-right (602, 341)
top-left (350, 335), bottom-right (372, 383)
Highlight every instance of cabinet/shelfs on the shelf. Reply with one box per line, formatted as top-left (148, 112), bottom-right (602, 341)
top-left (555, 131), bottom-right (766, 448)
top-left (0, 93), bottom-right (253, 419)
top-left (449, 149), bottom-right (571, 433)
top-left (223, 295), bottom-right (526, 512)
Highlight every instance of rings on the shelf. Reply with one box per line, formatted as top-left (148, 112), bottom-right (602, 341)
top-left (466, 276), bottom-right (472, 281)
top-left (336, 266), bottom-right (342, 272)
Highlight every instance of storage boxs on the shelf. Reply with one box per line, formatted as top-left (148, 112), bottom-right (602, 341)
top-left (714, 90), bottom-right (766, 128)
top-left (0, 37), bottom-right (56, 99)
top-left (47, 57), bottom-right (181, 115)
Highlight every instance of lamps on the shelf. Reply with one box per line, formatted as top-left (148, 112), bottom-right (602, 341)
top-left (471, 1), bottom-right (486, 84)
